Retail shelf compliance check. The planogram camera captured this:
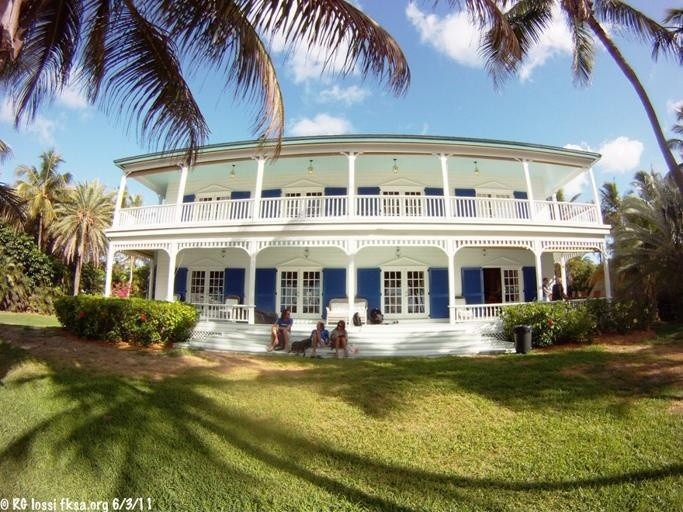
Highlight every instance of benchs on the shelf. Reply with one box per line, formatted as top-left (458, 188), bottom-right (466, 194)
top-left (324, 299), bottom-right (367, 326)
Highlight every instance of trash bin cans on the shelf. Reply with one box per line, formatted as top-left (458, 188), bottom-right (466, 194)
top-left (513, 324), bottom-right (532, 354)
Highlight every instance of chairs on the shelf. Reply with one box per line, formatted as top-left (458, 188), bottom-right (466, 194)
top-left (451, 295), bottom-right (472, 317)
top-left (218, 294), bottom-right (240, 319)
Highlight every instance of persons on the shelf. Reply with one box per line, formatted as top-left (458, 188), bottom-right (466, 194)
top-left (267, 308), bottom-right (293, 353)
top-left (329, 320), bottom-right (350, 359)
top-left (552, 274), bottom-right (568, 301)
top-left (308, 318), bottom-right (330, 358)
top-left (542, 277), bottom-right (551, 303)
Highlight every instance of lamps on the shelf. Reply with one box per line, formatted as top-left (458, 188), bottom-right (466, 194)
top-left (392, 158), bottom-right (398, 175)
top-left (229, 164), bottom-right (236, 177)
top-left (473, 160), bottom-right (479, 175)
top-left (306, 159), bottom-right (314, 175)
